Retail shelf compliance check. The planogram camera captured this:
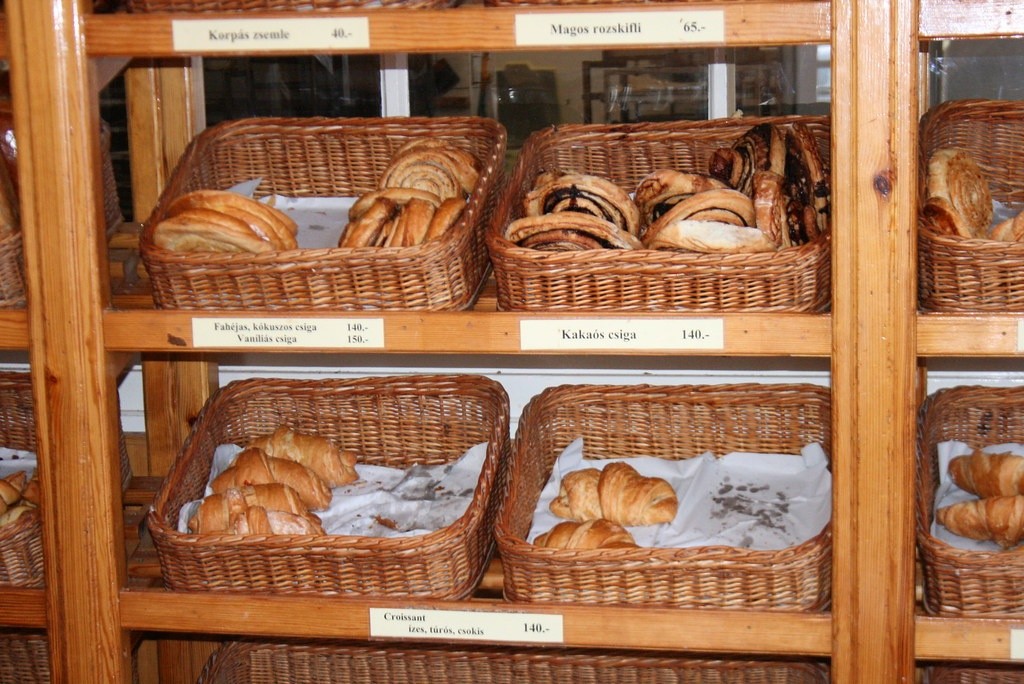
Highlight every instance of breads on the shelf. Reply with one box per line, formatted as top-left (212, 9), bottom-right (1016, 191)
top-left (187, 426), bottom-right (359, 536)
top-left (336, 136), bottom-right (481, 246)
top-left (149, 189), bottom-right (298, 255)
top-left (933, 449), bottom-right (1024, 550)
top-left (499, 123), bottom-right (827, 256)
top-left (0, 467), bottom-right (38, 528)
top-left (927, 145), bottom-right (1024, 245)
top-left (0, 68), bottom-right (25, 241)
top-left (549, 462), bottom-right (677, 527)
top-left (533, 517), bottom-right (643, 549)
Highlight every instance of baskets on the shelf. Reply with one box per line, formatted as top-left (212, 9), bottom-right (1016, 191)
top-left (923, 665), bottom-right (1023, 684)
top-left (146, 375), bottom-right (510, 601)
top-left (198, 639), bottom-right (831, 684)
top-left (0, 370), bottom-right (132, 589)
top-left (136, 114), bottom-right (508, 313)
top-left (487, 112), bottom-right (833, 313)
top-left (918, 98), bottom-right (1024, 317)
top-left (0, 97), bottom-right (123, 308)
top-left (494, 383), bottom-right (832, 613)
top-left (917, 383), bottom-right (1024, 618)
top-left (2, 634), bottom-right (51, 684)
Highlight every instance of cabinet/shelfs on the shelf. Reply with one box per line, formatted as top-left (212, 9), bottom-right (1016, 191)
top-left (0, 1), bottom-right (1024, 684)
